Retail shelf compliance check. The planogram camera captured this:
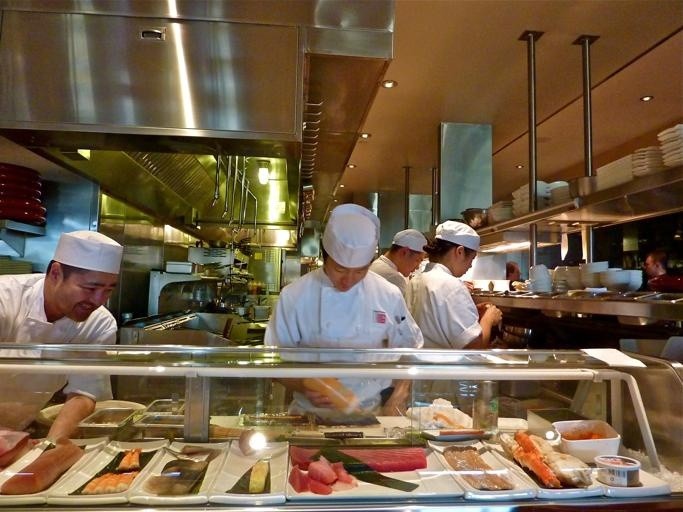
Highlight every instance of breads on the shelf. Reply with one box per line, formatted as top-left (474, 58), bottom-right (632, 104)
top-left (0, 444), bottom-right (84, 493)
top-left (501, 431), bottom-right (593, 488)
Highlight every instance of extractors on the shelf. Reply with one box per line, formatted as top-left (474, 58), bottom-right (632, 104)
top-left (119, 151), bottom-right (216, 209)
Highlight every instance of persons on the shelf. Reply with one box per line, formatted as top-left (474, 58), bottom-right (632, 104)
top-left (0, 228), bottom-right (125, 439)
top-left (506, 259), bottom-right (521, 282)
top-left (410, 218), bottom-right (504, 408)
top-left (369, 226), bottom-right (428, 301)
top-left (645, 250), bottom-right (682, 293)
top-left (262, 203), bottom-right (426, 416)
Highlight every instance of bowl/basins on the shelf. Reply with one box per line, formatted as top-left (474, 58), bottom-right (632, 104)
top-left (499, 322), bottom-right (535, 349)
top-left (235, 307), bottom-right (246, 316)
top-left (208, 239), bottom-right (227, 249)
top-left (527, 260), bottom-right (643, 296)
top-left (551, 419), bottom-right (621, 466)
top-left (459, 207), bottom-right (488, 230)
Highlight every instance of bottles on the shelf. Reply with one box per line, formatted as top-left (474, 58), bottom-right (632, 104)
top-left (246, 280), bottom-right (267, 296)
top-left (471, 380), bottom-right (499, 437)
top-left (301, 377), bottom-right (363, 416)
top-left (247, 304), bottom-right (254, 320)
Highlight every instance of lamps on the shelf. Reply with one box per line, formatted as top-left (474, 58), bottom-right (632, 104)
top-left (255, 161), bottom-right (274, 184)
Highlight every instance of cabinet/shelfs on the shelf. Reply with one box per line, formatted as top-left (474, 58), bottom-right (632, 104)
top-left (468, 167), bottom-right (681, 321)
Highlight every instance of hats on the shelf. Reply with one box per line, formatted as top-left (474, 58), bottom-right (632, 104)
top-left (322, 203), bottom-right (380, 269)
top-left (52, 230), bottom-right (124, 275)
top-left (391, 229), bottom-right (428, 253)
top-left (434, 220), bottom-right (480, 252)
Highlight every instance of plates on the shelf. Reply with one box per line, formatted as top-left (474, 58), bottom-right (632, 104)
top-left (645, 274), bottom-right (683, 294)
top-left (206, 440), bottom-right (289, 506)
top-left (0, 257), bottom-right (32, 275)
top-left (0, 160), bottom-right (47, 224)
top-left (126, 439), bottom-right (230, 506)
top-left (268, 291), bottom-right (279, 295)
top-left (45, 437), bottom-right (170, 505)
top-left (0, 436), bottom-right (110, 506)
top-left (493, 415), bottom-right (528, 434)
top-left (285, 444), bottom-right (465, 502)
top-left (482, 440), bottom-right (604, 500)
top-left (35, 396), bottom-right (146, 438)
top-left (426, 438), bottom-right (537, 502)
top-left (591, 467), bottom-right (671, 500)
top-left (488, 122), bottom-right (682, 224)
